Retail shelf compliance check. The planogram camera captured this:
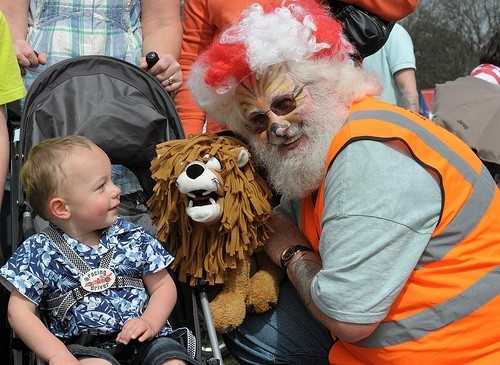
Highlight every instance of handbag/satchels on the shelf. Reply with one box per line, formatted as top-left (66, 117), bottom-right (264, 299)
top-left (333, 3), bottom-right (396, 59)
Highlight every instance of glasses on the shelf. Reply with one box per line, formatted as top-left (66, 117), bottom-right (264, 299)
top-left (243, 82), bottom-right (309, 135)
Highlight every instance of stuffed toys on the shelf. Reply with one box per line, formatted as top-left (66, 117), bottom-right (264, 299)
top-left (146, 134), bottom-right (278, 332)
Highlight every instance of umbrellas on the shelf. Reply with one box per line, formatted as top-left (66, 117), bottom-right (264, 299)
top-left (431, 76), bottom-right (500, 164)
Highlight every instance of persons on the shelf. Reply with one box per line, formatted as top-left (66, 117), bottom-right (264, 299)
top-left (0, 136), bottom-right (201, 365)
top-left (174, 0), bottom-right (283, 354)
top-left (190, 5), bottom-right (500, 365)
top-left (363, 22), bottom-right (419, 114)
top-left (0, 10), bottom-right (27, 210)
top-left (5, 0), bottom-right (183, 239)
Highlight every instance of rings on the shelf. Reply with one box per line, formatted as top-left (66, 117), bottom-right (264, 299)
top-left (168, 76), bottom-right (175, 85)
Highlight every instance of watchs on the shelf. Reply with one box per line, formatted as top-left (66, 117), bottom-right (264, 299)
top-left (280, 245), bottom-right (315, 268)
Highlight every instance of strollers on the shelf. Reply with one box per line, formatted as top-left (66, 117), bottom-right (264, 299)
top-left (9, 48), bottom-right (226, 365)
